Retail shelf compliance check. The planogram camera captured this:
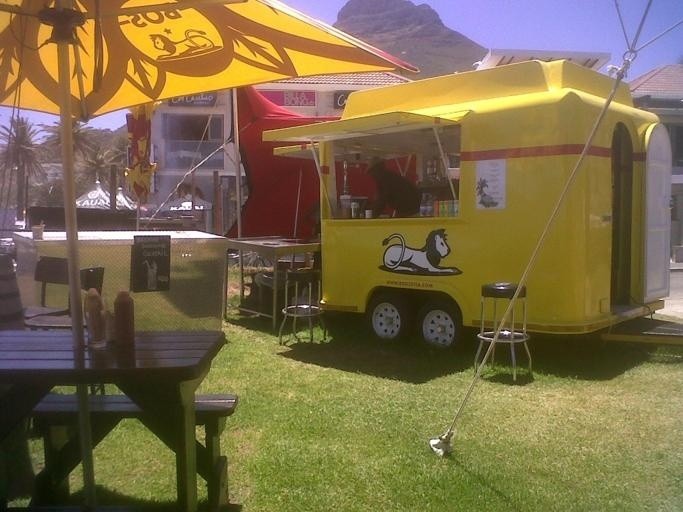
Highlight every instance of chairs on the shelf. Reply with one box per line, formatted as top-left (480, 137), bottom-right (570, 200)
top-left (23, 255), bottom-right (72, 318)
top-left (25, 267), bottom-right (104, 331)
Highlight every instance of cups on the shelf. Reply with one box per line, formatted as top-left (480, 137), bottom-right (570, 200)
top-left (29, 224), bottom-right (45, 241)
top-left (364, 210), bottom-right (373, 220)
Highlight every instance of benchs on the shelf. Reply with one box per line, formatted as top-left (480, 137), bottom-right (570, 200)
top-left (32, 393), bottom-right (239, 507)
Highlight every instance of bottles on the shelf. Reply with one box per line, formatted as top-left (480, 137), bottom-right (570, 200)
top-left (83, 287), bottom-right (107, 350)
top-left (419, 198), bottom-right (459, 218)
top-left (114, 289), bottom-right (136, 347)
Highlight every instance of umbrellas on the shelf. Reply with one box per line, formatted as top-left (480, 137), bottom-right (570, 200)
top-left (0, 0), bottom-right (421, 512)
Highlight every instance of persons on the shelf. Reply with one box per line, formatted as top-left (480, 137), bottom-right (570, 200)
top-left (364, 154), bottom-right (421, 219)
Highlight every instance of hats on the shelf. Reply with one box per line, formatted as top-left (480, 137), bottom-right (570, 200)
top-left (365, 156), bottom-right (385, 175)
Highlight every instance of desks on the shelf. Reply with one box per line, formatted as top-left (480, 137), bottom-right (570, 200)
top-left (227, 235), bottom-right (321, 329)
top-left (0, 330), bottom-right (228, 512)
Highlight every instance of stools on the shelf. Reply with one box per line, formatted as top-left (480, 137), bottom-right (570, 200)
top-left (474, 281), bottom-right (532, 382)
top-left (279, 268), bottom-right (325, 346)
